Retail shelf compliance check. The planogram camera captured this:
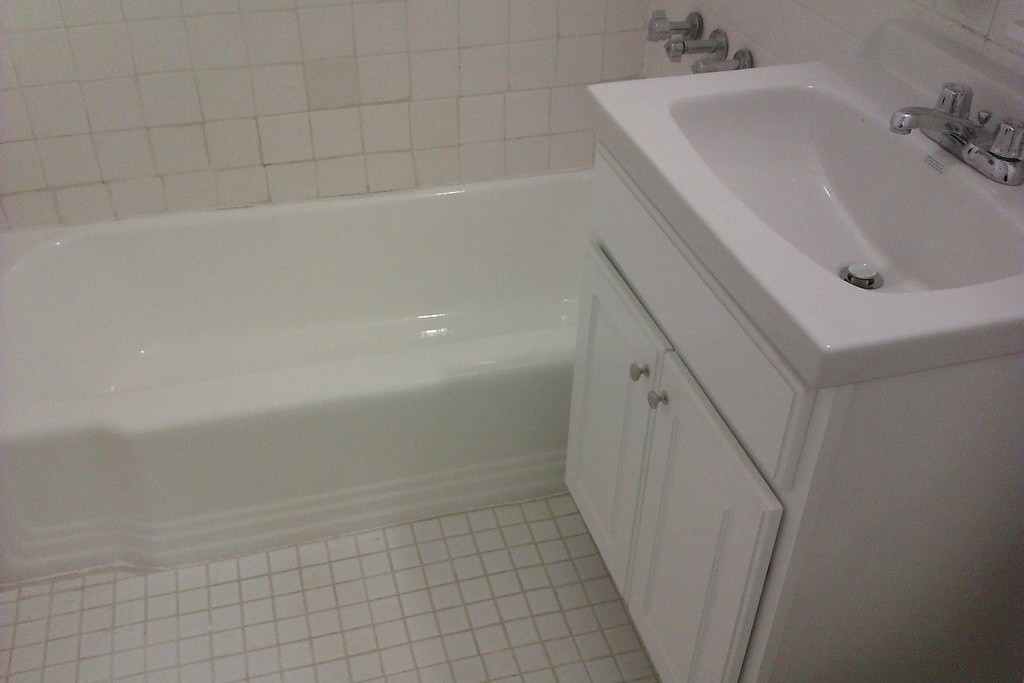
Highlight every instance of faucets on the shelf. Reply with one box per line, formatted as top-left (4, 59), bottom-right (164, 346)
top-left (888, 82), bottom-right (1023, 187)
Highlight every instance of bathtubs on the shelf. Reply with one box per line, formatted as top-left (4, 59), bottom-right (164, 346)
top-left (1, 168), bottom-right (595, 587)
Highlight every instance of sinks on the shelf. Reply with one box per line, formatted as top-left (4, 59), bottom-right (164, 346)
top-left (586, 60), bottom-right (1023, 389)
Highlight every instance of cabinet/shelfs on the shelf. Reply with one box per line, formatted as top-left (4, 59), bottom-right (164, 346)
top-left (564, 138), bottom-right (1024, 683)
